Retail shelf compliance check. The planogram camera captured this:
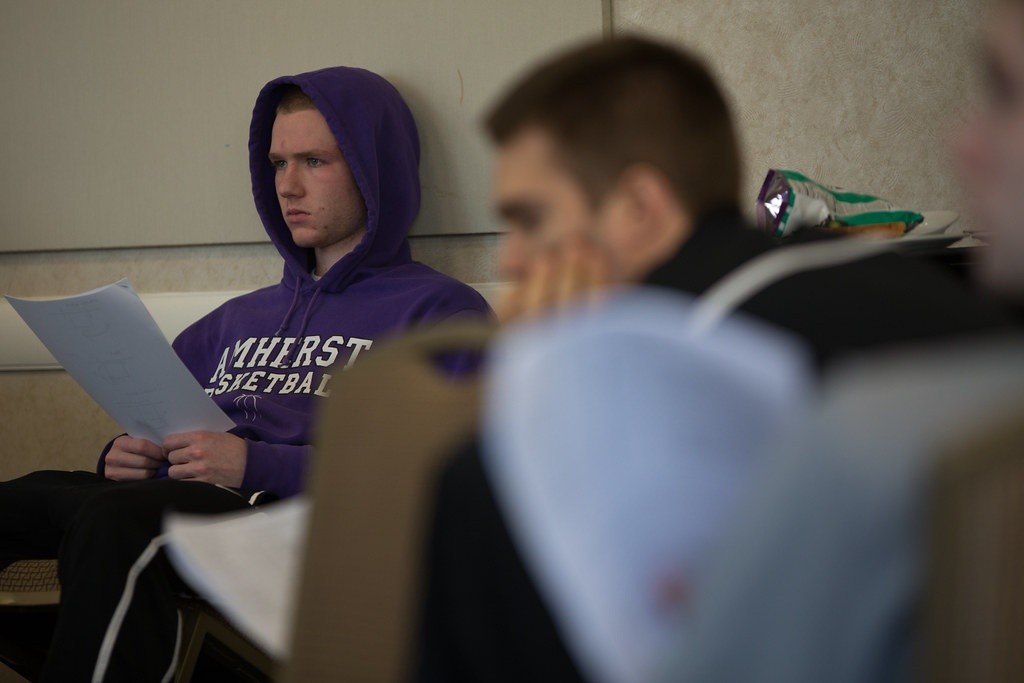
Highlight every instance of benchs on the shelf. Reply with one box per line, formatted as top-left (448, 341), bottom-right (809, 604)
top-left (0, 277), bottom-right (529, 607)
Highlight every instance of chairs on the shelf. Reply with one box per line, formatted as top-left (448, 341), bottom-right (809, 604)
top-left (290, 323), bottom-right (505, 680)
top-left (921, 414), bottom-right (1024, 683)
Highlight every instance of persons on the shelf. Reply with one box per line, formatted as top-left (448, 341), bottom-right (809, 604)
top-left (399, 33), bottom-right (970, 683)
top-left (0, 66), bottom-right (501, 683)
top-left (906, 0), bottom-right (1024, 683)
